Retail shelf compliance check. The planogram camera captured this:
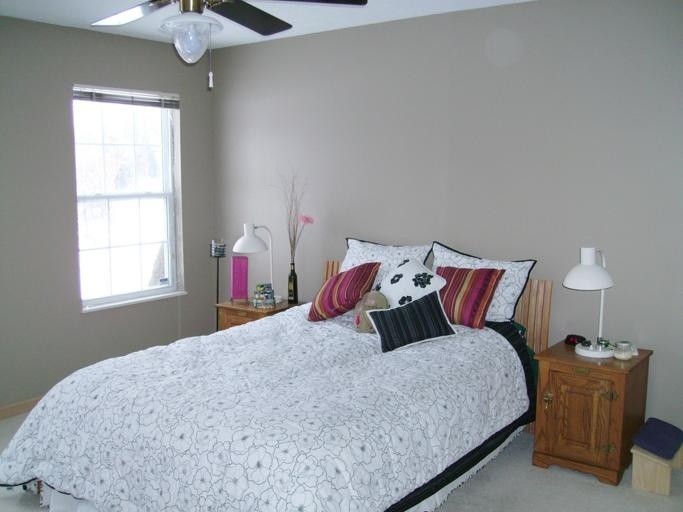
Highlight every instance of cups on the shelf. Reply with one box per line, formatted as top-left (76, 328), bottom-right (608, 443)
top-left (599, 338), bottom-right (610, 348)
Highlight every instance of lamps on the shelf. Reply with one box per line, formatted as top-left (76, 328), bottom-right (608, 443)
top-left (558, 246), bottom-right (617, 359)
top-left (160, 0), bottom-right (221, 64)
top-left (233, 221), bottom-right (282, 303)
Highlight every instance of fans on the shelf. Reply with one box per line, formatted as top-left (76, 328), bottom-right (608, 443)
top-left (88, 0), bottom-right (368, 36)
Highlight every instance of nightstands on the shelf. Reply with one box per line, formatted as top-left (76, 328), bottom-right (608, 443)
top-left (529, 338), bottom-right (652, 486)
top-left (212, 298), bottom-right (304, 330)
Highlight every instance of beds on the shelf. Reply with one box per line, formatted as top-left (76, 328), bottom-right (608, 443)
top-left (1, 260), bottom-right (554, 512)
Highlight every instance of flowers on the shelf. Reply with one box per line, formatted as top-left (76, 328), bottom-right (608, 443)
top-left (274, 174), bottom-right (315, 263)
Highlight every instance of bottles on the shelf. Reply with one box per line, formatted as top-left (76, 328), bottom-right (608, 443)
top-left (612, 340), bottom-right (633, 361)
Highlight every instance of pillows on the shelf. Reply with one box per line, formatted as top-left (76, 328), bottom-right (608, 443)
top-left (307, 262), bottom-right (379, 323)
top-left (365, 291), bottom-right (456, 354)
top-left (436, 264), bottom-right (505, 329)
top-left (371, 253), bottom-right (446, 312)
top-left (339, 240), bottom-right (433, 288)
top-left (430, 241), bottom-right (538, 324)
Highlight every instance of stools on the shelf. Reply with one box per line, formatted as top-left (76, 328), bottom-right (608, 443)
top-left (631, 413), bottom-right (682, 496)
